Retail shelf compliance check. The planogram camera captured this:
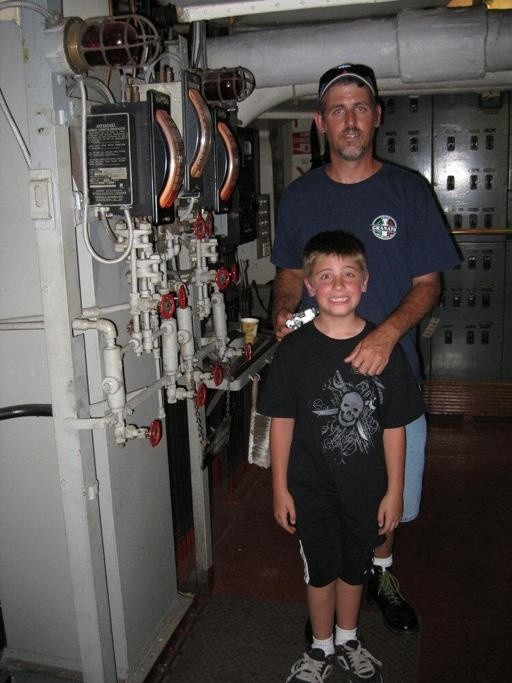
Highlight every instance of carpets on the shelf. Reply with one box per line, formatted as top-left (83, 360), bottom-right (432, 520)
top-left (155, 592), bottom-right (425, 683)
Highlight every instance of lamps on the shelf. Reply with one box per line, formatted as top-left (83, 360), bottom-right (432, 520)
top-left (202, 65), bottom-right (258, 107)
top-left (61, 12), bottom-right (165, 75)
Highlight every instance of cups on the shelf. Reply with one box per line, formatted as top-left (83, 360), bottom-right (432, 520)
top-left (240, 318), bottom-right (260, 345)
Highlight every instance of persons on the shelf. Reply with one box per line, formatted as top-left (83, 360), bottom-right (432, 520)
top-left (258, 231), bottom-right (427, 683)
top-left (273, 63), bottom-right (462, 645)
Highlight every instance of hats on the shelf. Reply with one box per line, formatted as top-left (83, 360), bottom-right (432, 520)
top-left (318, 62), bottom-right (380, 102)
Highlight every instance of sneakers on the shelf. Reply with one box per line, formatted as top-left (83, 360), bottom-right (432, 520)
top-left (371, 567), bottom-right (419, 634)
top-left (286, 649), bottom-right (338, 683)
top-left (335, 641), bottom-right (385, 681)
